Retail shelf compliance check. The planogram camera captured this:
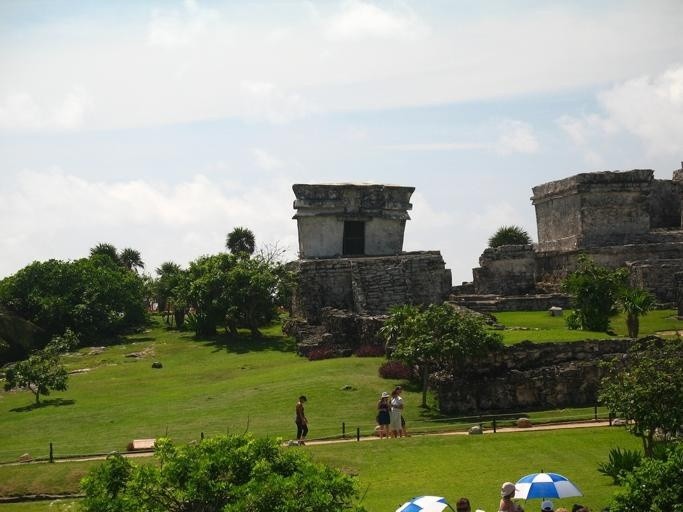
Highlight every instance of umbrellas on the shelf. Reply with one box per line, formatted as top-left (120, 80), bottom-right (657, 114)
top-left (512, 469), bottom-right (585, 501)
top-left (395, 495), bottom-right (448, 512)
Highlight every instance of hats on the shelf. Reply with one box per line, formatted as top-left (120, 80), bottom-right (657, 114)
top-left (500, 482), bottom-right (515, 498)
top-left (381, 392), bottom-right (390, 398)
top-left (540, 500), bottom-right (554, 511)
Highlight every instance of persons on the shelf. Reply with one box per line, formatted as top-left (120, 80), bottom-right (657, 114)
top-left (294, 395), bottom-right (309, 444)
top-left (498, 482), bottom-right (520, 512)
top-left (388, 392), bottom-right (404, 439)
top-left (540, 501), bottom-right (555, 512)
top-left (455, 498), bottom-right (471, 512)
top-left (375, 391), bottom-right (390, 439)
top-left (571, 503), bottom-right (585, 512)
top-left (389, 385), bottom-right (407, 438)
